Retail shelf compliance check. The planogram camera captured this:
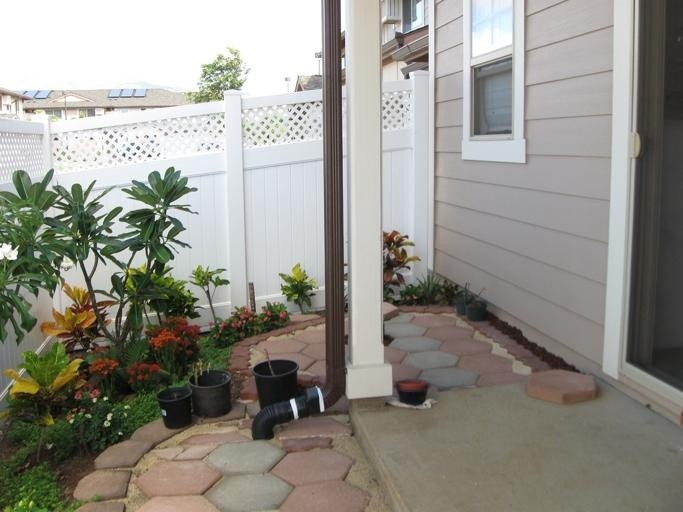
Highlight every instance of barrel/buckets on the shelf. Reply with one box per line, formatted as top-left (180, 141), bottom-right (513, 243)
top-left (251, 359), bottom-right (299, 410)
top-left (188, 369), bottom-right (232, 417)
top-left (155, 371), bottom-right (194, 429)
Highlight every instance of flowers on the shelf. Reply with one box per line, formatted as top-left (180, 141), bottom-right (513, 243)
top-left (208, 300), bottom-right (289, 347)
top-left (62, 314), bottom-right (202, 439)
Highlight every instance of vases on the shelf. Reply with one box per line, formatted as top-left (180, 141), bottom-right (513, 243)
top-left (395, 379), bottom-right (430, 404)
top-left (155, 358), bottom-right (299, 430)
top-left (454, 290), bottom-right (487, 322)
